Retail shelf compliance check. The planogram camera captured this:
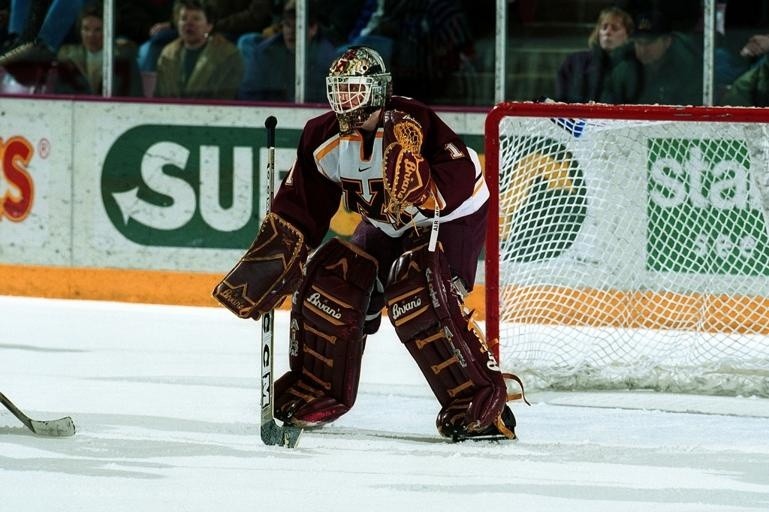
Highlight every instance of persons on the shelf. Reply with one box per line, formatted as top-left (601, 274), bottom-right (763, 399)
top-left (1, 1), bottom-right (481, 107)
top-left (213, 47), bottom-right (507, 438)
top-left (555, 0), bottom-right (769, 107)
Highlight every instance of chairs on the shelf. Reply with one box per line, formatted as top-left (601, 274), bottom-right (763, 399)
top-left (136, 24), bottom-right (391, 83)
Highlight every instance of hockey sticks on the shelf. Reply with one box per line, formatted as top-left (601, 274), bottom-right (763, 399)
top-left (0, 392), bottom-right (76, 436)
top-left (259, 115), bottom-right (304, 448)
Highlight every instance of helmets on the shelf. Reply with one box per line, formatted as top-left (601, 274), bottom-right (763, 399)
top-left (326, 46), bottom-right (392, 108)
